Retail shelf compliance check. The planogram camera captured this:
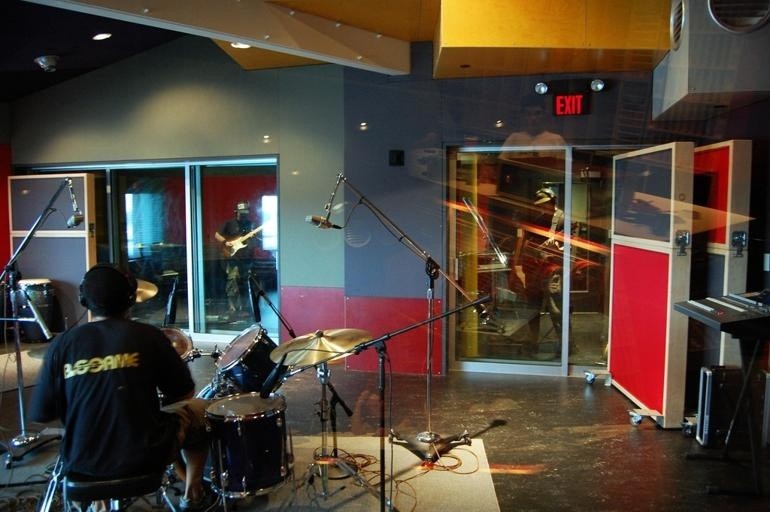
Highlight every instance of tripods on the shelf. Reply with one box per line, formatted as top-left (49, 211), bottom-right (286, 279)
top-left (278, 399), bottom-right (394, 512)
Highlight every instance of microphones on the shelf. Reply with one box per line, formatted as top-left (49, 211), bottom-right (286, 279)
top-left (24, 296), bottom-right (53, 340)
top-left (260, 353), bottom-right (286, 399)
top-left (164, 280), bottom-right (179, 324)
top-left (304, 215), bottom-right (342, 231)
top-left (248, 278), bottom-right (261, 323)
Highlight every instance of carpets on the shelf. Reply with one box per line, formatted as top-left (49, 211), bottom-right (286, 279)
top-left (0, 339), bottom-right (48, 392)
top-left (0, 436), bottom-right (500, 512)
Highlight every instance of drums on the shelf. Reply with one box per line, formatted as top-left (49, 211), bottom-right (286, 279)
top-left (216, 323), bottom-right (289, 392)
top-left (205, 393), bottom-right (288, 497)
top-left (160, 325), bottom-right (194, 364)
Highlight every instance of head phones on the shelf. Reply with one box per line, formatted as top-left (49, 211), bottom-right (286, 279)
top-left (78, 263), bottom-right (138, 308)
top-left (534, 188), bottom-right (558, 204)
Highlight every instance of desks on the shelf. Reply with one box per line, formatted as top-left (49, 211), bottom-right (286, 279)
top-left (133, 244), bottom-right (186, 275)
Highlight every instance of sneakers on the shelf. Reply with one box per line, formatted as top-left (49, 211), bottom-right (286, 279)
top-left (556, 344), bottom-right (578, 357)
top-left (178, 487), bottom-right (228, 512)
top-left (517, 343), bottom-right (538, 354)
top-left (67, 503), bottom-right (117, 512)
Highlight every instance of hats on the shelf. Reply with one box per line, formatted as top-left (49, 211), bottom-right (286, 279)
top-left (534, 187), bottom-right (555, 205)
top-left (84, 264), bottom-right (139, 312)
top-left (236, 202), bottom-right (250, 215)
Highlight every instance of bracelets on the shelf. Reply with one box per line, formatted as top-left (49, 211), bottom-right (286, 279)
top-left (222, 240), bottom-right (227, 245)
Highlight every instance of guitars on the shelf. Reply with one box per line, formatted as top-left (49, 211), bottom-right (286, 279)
top-left (223, 220), bottom-right (265, 257)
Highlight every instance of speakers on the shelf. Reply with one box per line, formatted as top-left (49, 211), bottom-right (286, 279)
top-left (695, 364), bottom-right (766, 450)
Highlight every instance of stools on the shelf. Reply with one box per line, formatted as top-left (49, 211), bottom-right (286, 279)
top-left (59, 462), bottom-right (162, 512)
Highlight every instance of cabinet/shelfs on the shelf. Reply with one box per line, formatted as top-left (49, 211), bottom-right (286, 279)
top-left (459, 253), bottom-right (511, 272)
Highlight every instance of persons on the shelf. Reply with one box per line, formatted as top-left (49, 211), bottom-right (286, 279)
top-left (510, 187), bottom-right (578, 358)
top-left (215, 199), bottom-right (256, 315)
top-left (29, 264), bottom-right (222, 512)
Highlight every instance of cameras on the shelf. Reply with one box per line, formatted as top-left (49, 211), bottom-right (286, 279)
top-left (66, 213), bottom-right (84, 229)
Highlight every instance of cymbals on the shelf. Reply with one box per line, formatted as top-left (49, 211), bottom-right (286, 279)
top-left (134, 279), bottom-right (160, 302)
top-left (270, 328), bottom-right (372, 366)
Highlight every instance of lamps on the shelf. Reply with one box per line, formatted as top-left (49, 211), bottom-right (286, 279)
top-left (535, 82), bottom-right (552, 95)
top-left (591, 79), bottom-right (606, 92)
top-left (34, 55), bottom-right (59, 72)
